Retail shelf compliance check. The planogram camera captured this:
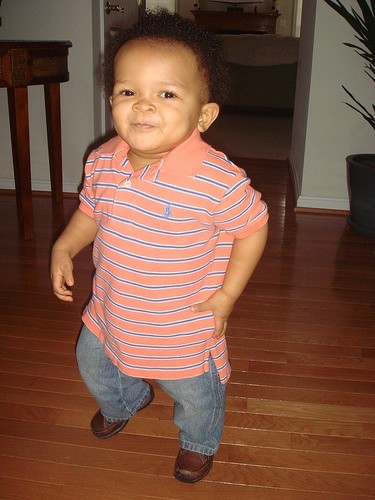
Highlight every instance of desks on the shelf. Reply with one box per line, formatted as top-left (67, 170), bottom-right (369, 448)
top-left (0, 37), bottom-right (73, 241)
top-left (190, 9), bottom-right (281, 34)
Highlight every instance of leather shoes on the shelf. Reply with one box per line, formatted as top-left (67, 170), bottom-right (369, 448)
top-left (89, 379), bottom-right (156, 439)
top-left (173, 447), bottom-right (217, 483)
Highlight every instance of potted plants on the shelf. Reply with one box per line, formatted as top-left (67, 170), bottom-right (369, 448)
top-left (324, 0), bottom-right (375, 229)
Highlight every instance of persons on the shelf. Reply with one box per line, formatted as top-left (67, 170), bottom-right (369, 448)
top-left (50, 7), bottom-right (269, 483)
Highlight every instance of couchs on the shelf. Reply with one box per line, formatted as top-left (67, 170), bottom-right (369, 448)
top-left (215, 35), bottom-right (299, 110)
